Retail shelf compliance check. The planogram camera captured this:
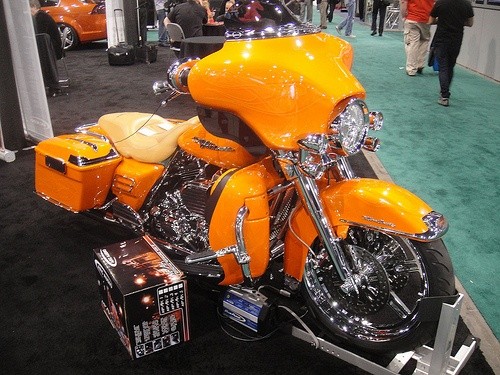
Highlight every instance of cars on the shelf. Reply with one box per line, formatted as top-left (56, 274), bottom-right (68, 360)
top-left (31, 1), bottom-right (106, 50)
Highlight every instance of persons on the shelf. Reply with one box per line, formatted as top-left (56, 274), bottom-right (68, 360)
top-left (30, 0), bottom-right (63, 60)
top-left (153, 0), bottom-right (475, 106)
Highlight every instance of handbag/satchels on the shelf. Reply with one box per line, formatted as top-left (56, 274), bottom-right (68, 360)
top-left (381, 0), bottom-right (389, 6)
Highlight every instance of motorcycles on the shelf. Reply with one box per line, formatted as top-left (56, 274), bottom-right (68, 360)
top-left (32, 0), bottom-right (455, 352)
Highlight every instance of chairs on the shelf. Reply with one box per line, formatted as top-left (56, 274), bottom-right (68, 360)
top-left (162, 23), bottom-right (185, 71)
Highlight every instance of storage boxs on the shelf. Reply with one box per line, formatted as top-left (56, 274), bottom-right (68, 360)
top-left (91, 235), bottom-right (191, 361)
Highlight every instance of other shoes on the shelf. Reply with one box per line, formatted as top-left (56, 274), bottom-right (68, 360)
top-left (437, 97), bottom-right (448, 105)
top-left (418, 69), bottom-right (422, 73)
top-left (408, 72), bottom-right (415, 75)
top-left (336, 26), bottom-right (341, 34)
top-left (345, 35), bottom-right (356, 38)
top-left (379, 32), bottom-right (382, 36)
top-left (371, 32), bottom-right (377, 35)
top-left (318, 25), bottom-right (327, 29)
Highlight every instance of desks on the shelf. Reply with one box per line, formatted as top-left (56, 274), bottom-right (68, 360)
top-left (179, 36), bottom-right (225, 59)
